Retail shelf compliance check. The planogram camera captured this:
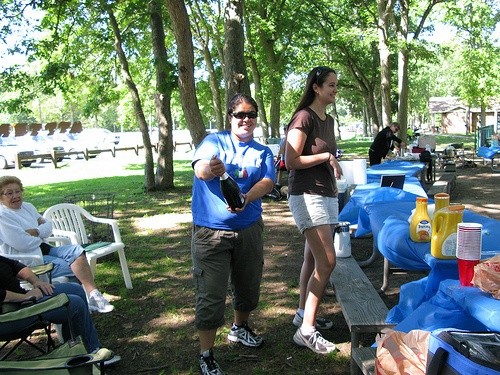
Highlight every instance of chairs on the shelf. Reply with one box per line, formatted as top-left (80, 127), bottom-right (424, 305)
top-left (40, 201), bottom-right (133, 292)
top-left (0, 236), bottom-right (115, 375)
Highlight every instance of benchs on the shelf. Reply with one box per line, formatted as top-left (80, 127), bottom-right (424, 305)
top-left (425, 180), bottom-right (450, 199)
top-left (444, 162), bottom-right (457, 173)
top-left (351, 346), bottom-right (377, 375)
top-left (328, 246), bottom-right (398, 375)
top-left (438, 174), bottom-right (455, 184)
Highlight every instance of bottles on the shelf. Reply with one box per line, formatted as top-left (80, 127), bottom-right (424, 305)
top-left (426, 144), bottom-right (430, 151)
top-left (433, 192), bottom-right (450, 214)
top-left (430, 205), bottom-right (465, 259)
top-left (410, 196), bottom-right (432, 243)
top-left (213, 156), bottom-right (245, 212)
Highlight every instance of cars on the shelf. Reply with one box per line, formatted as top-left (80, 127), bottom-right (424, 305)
top-left (0, 127), bottom-right (123, 169)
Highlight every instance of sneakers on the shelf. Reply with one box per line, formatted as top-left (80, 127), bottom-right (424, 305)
top-left (293, 311), bottom-right (333, 330)
top-left (198, 348), bottom-right (225, 375)
top-left (97, 354), bottom-right (121, 366)
top-left (293, 326), bottom-right (336, 354)
top-left (103, 292), bottom-right (121, 302)
top-left (227, 320), bottom-right (263, 347)
top-left (88, 289), bottom-right (115, 313)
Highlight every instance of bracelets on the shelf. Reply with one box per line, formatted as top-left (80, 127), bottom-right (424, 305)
top-left (327, 152), bottom-right (331, 161)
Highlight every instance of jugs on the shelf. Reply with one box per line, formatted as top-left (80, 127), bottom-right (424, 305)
top-left (334, 225), bottom-right (352, 258)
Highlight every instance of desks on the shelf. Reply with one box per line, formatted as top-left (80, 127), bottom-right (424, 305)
top-left (337, 143), bottom-right (500, 348)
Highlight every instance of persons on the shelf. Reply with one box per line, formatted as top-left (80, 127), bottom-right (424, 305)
top-left (0, 175), bottom-right (116, 314)
top-left (190, 95), bottom-right (276, 375)
top-left (276, 125), bottom-right (288, 184)
top-left (369, 122), bottom-right (406, 166)
top-left (0, 256), bottom-right (121, 364)
top-left (284, 66), bottom-right (344, 353)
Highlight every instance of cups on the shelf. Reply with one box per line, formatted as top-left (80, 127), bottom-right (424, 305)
top-left (456, 222), bottom-right (483, 287)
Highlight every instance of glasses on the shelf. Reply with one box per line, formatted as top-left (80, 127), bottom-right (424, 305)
top-left (314, 67), bottom-right (329, 82)
top-left (2, 189), bottom-right (22, 196)
top-left (394, 126), bottom-right (399, 131)
top-left (231, 111), bottom-right (258, 119)
